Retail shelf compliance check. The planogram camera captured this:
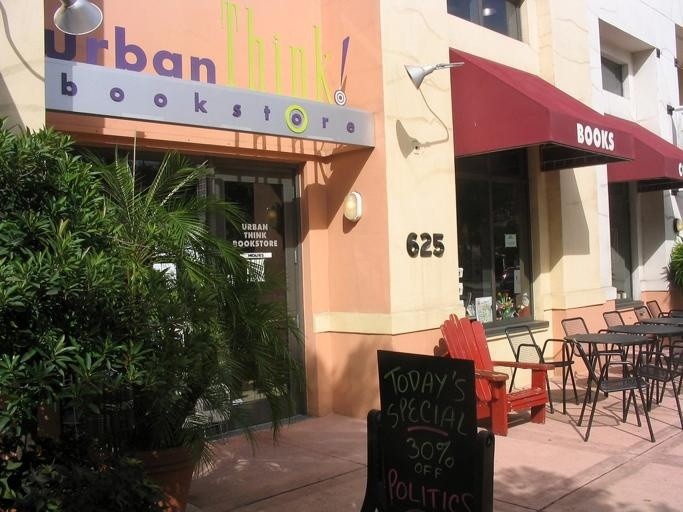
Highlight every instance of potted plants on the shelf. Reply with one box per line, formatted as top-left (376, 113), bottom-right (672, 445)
top-left (81, 131), bottom-right (311, 511)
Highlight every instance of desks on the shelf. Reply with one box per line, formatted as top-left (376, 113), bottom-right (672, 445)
top-left (640, 317), bottom-right (683, 357)
top-left (564, 333), bottom-right (647, 422)
top-left (607, 325), bottom-right (682, 396)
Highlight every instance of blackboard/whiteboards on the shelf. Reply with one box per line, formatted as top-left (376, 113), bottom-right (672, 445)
top-left (376, 349), bottom-right (481, 512)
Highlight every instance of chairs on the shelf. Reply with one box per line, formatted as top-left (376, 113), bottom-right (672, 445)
top-left (572, 338), bottom-right (655, 442)
top-left (561, 318), bottom-right (606, 405)
top-left (603, 311), bottom-right (631, 377)
top-left (671, 340), bottom-right (683, 371)
top-left (634, 306), bottom-right (666, 363)
top-left (435, 314), bottom-right (555, 436)
top-left (646, 300), bottom-right (682, 352)
top-left (625, 344), bottom-right (682, 430)
top-left (505, 325), bottom-right (579, 413)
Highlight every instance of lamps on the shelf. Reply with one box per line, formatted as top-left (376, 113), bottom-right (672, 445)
top-left (403, 61), bottom-right (466, 153)
top-left (53, 1), bottom-right (102, 36)
top-left (344, 192), bottom-right (363, 222)
